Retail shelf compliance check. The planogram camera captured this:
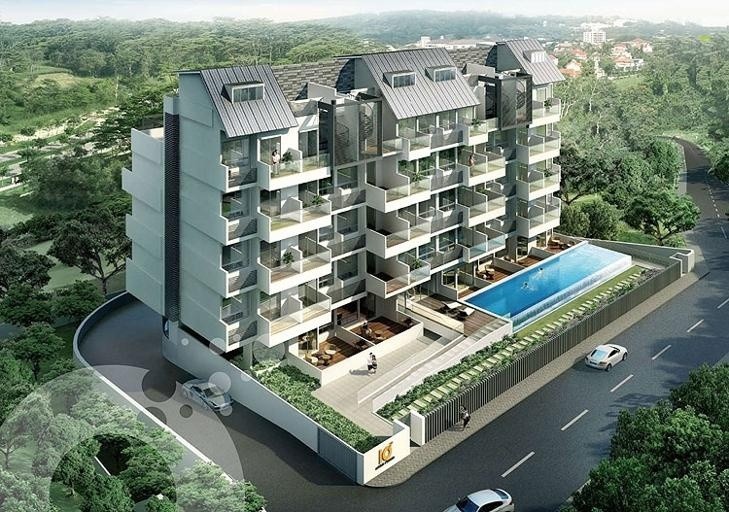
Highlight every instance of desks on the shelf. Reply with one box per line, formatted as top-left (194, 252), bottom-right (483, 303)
top-left (374, 330), bottom-right (382, 337)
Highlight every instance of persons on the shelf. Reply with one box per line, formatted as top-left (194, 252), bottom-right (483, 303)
top-left (271, 151), bottom-right (278, 176)
top-left (522, 282), bottom-right (529, 290)
top-left (460, 407), bottom-right (472, 432)
top-left (275, 150), bottom-right (281, 177)
top-left (372, 355), bottom-right (378, 374)
top-left (468, 153), bottom-right (477, 177)
top-left (367, 352), bottom-right (373, 376)
top-left (363, 322), bottom-right (371, 337)
top-left (539, 267), bottom-right (544, 273)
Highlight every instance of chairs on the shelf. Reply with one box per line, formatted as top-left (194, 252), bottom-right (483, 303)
top-left (305, 343), bottom-right (336, 367)
top-left (550, 234), bottom-right (575, 250)
top-left (477, 265), bottom-right (495, 278)
top-left (441, 301), bottom-right (475, 321)
top-left (360, 325), bottom-right (372, 339)
